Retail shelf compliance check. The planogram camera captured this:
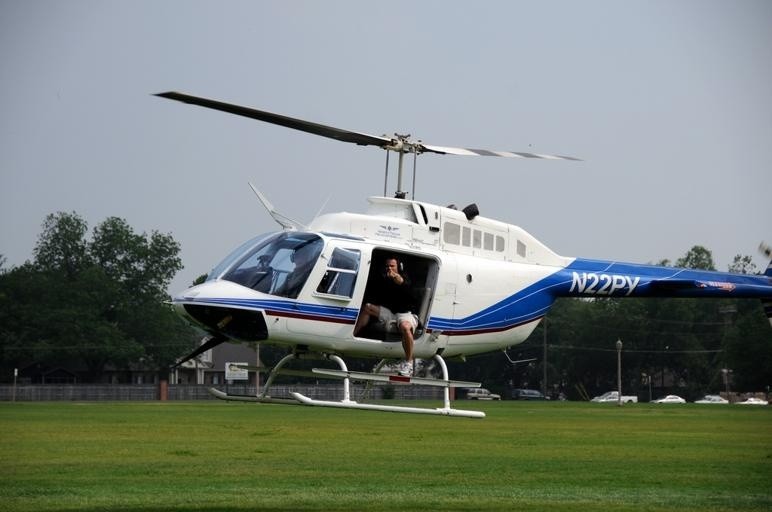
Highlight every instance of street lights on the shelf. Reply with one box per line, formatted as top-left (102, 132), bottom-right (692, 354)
top-left (614, 338), bottom-right (623, 408)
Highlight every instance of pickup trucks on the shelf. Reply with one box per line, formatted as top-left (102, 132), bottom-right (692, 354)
top-left (592, 390), bottom-right (639, 405)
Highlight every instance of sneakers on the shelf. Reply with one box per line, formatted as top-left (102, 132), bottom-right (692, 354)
top-left (398, 362), bottom-right (414, 377)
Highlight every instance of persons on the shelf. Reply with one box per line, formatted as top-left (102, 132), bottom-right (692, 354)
top-left (353, 256), bottom-right (420, 377)
top-left (274, 244), bottom-right (313, 300)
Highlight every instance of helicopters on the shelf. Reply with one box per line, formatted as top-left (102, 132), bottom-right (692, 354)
top-left (152, 88), bottom-right (772, 418)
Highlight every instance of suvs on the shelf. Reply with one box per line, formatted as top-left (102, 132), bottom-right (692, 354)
top-left (512, 389), bottom-right (550, 399)
top-left (459, 387), bottom-right (502, 401)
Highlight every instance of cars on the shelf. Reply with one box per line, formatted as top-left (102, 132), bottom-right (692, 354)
top-left (734, 396), bottom-right (767, 406)
top-left (650, 393), bottom-right (688, 404)
top-left (693, 394), bottom-right (729, 404)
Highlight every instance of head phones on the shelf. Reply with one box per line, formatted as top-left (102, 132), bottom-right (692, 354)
top-left (384, 256), bottom-right (404, 272)
top-left (290, 245), bottom-right (313, 262)
